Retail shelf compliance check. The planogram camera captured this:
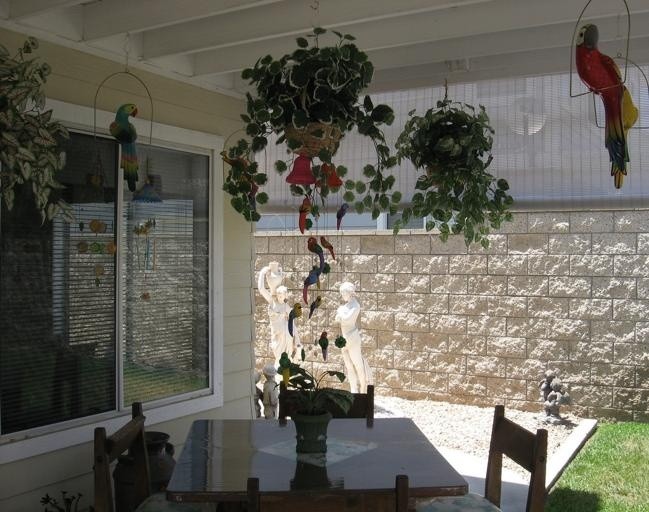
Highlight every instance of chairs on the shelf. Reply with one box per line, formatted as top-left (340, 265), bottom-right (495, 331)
top-left (269, 385), bottom-right (383, 424)
top-left (413, 400), bottom-right (549, 510)
top-left (240, 472), bottom-right (410, 511)
top-left (91, 402), bottom-right (175, 511)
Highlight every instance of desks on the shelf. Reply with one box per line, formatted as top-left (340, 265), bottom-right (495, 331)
top-left (169, 415), bottom-right (475, 510)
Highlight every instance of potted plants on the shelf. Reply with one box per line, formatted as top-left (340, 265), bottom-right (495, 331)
top-left (221, 26), bottom-right (390, 248)
top-left (396, 78), bottom-right (517, 255)
top-left (274, 363), bottom-right (355, 444)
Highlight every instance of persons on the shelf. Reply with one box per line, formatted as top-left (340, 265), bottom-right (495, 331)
top-left (542, 379), bottom-right (564, 418)
top-left (258, 267), bottom-right (301, 383)
top-left (262, 366), bottom-right (278, 419)
top-left (254, 369), bottom-right (264, 419)
top-left (538, 368), bottom-right (557, 418)
top-left (333, 282), bottom-right (367, 394)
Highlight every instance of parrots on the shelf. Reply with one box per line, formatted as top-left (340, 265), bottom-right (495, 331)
top-left (279, 197), bottom-right (350, 389)
top-left (575, 22), bottom-right (639, 189)
top-left (109, 103), bottom-right (139, 192)
